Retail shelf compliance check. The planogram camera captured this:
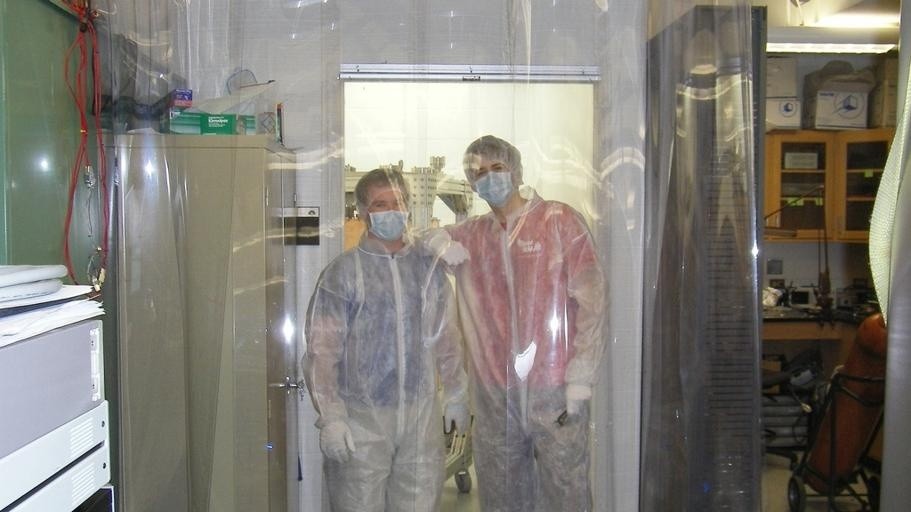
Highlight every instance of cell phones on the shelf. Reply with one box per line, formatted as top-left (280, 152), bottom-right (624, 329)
top-left (556, 409), bottom-right (568, 426)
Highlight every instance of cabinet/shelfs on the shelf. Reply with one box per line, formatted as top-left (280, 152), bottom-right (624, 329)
top-left (764, 126), bottom-right (897, 244)
top-left (117, 133), bottom-right (304, 512)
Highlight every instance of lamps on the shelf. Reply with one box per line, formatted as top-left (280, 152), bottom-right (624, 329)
top-left (766, 26), bottom-right (900, 54)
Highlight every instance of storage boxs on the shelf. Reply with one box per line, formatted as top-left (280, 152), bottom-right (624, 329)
top-left (766, 57), bottom-right (898, 130)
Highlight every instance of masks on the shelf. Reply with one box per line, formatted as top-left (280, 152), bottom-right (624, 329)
top-left (472, 172), bottom-right (515, 206)
top-left (366, 210), bottom-right (409, 241)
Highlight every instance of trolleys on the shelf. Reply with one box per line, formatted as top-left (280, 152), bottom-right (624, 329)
top-left (788, 368), bottom-right (885, 511)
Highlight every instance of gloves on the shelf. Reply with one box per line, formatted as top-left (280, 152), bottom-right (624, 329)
top-left (443, 404), bottom-right (471, 435)
top-left (319, 421), bottom-right (356, 466)
top-left (566, 385), bottom-right (592, 415)
top-left (431, 235), bottom-right (470, 267)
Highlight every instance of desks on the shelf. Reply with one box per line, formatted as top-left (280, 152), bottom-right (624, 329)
top-left (761, 305), bottom-right (882, 341)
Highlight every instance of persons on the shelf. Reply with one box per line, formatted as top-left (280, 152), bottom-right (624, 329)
top-left (415, 133), bottom-right (607, 511)
top-left (299, 168), bottom-right (474, 512)
